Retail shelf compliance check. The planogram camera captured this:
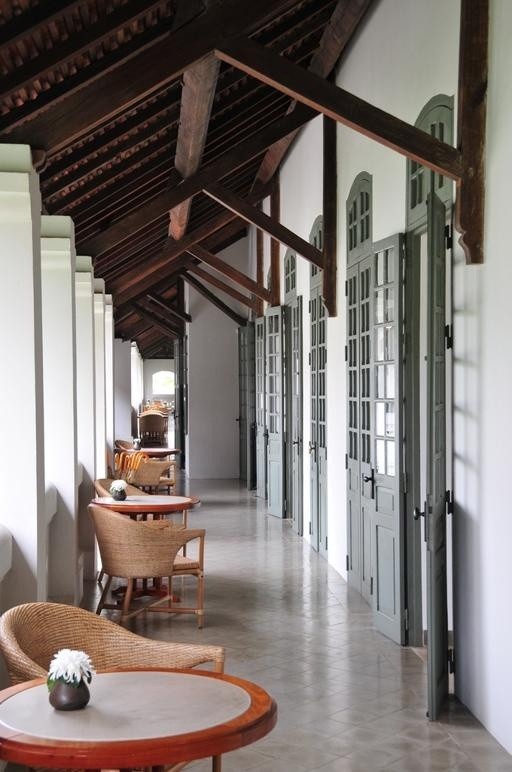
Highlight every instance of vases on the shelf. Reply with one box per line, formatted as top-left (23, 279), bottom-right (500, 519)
top-left (48, 673), bottom-right (90, 710)
top-left (112, 490), bottom-right (126, 501)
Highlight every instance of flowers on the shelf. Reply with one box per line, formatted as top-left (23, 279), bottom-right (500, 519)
top-left (44, 648), bottom-right (94, 686)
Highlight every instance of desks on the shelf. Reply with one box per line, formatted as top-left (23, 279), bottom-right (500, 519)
top-left (88, 495), bottom-right (201, 601)
top-left (0, 667), bottom-right (278, 771)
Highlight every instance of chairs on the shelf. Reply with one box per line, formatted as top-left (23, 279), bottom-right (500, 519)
top-left (93, 399), bottom-right (182, 499)
top-left (0, 602), bottom-right (228, 772)
top-left (89, 503), bottom-right (208, 631)
